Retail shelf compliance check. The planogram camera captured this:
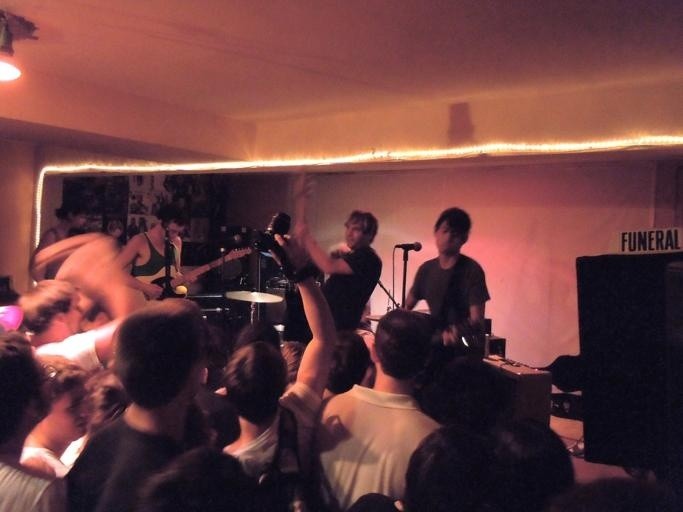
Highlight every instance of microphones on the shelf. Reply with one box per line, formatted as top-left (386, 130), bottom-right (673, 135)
top-left (162, 221), bottom-right (170, 240)
top-left (395, 242), bottom-right (422, 251)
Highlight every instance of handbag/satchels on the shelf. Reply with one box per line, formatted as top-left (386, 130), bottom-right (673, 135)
top-left (258, 409), bottom-right (340, 511)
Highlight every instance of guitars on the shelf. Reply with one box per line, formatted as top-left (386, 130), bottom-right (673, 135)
top-left (135, 246), bottom-right (252, 300)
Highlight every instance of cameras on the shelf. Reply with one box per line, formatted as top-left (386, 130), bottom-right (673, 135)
top-left (261, 211), bottom-right (291, 258)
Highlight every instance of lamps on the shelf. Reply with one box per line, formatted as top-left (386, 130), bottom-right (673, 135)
top-left (0, 14), bottom-right (35, 82)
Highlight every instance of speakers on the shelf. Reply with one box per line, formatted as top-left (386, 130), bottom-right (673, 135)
top-left (576, 254), bottom-right (683, 469)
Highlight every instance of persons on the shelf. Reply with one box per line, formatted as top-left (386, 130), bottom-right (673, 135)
top-left (350, 423), bottom-right (514, 510)
top-left (543, 477), bottom-right (680, 511)
top-left (0, 197), bottom-right (490, 510)
top-left (488, 416), bottom-right (577, 511)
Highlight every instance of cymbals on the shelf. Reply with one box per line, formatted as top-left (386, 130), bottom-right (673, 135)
top-left (365, 314), bottom-right (381, 321)
top-left (226, 290), bottom-right (283, 303)
